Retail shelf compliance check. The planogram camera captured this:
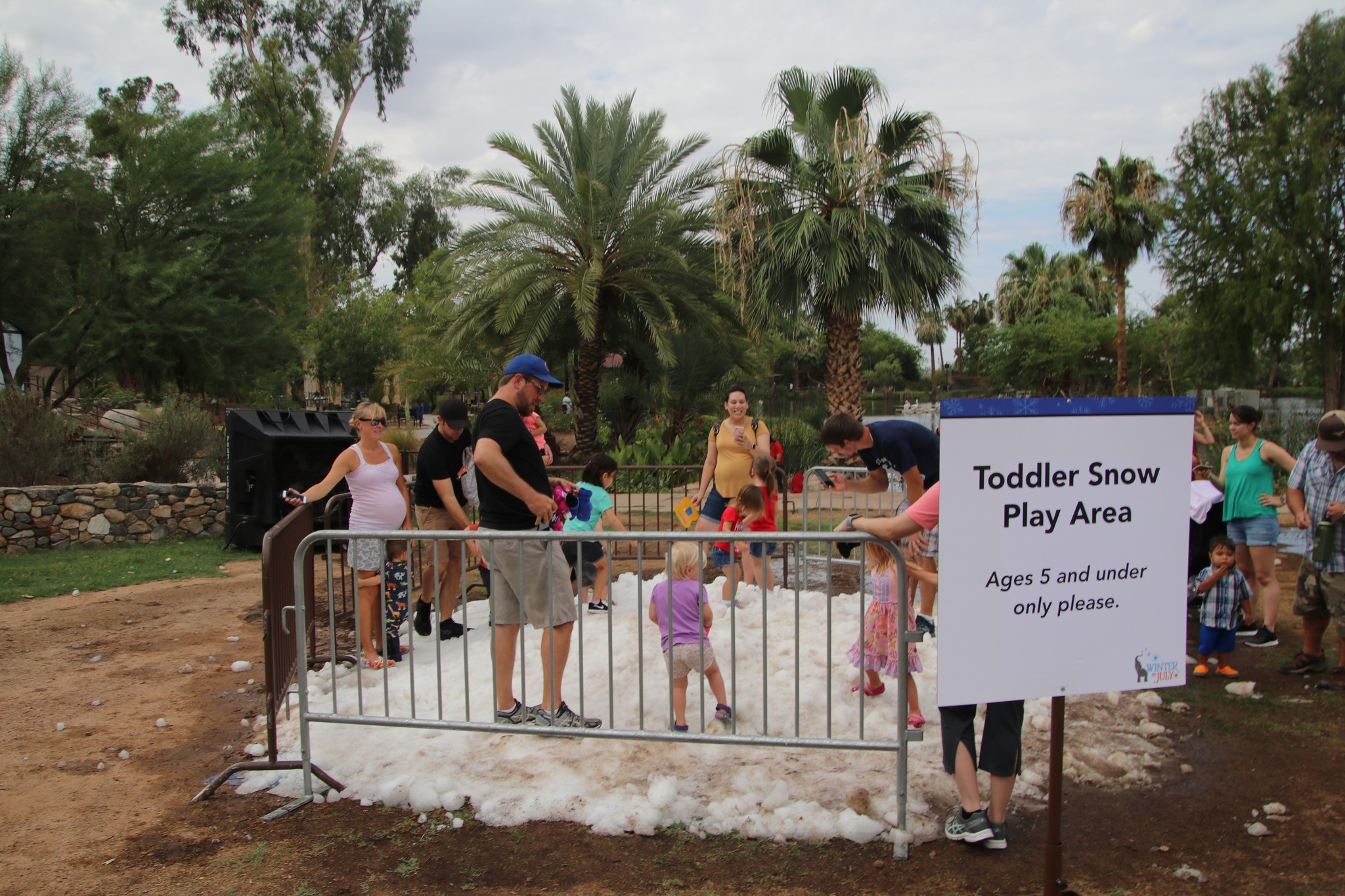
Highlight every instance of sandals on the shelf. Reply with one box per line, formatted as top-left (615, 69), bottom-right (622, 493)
top-left (668, 720), bottom-right (688, 733)
top-left (715, 703), bottom-right (732, 722)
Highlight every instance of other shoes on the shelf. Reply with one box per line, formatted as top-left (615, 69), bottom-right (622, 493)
top-left (399, 645), bottom-right (415, 655)
top-left (851, 682), bottom-right (885, 696)
top-left (361, 655), bottom-right (395, 670)
top-left (907, 713), bottom-right (925, 729)
top-left (1215, 666), bottom-right (1239, 677)
top-left (1279, 647), bottom-right (1326, 672)
top-left (1193, 665), bottom-right (1208, 677)
top-left (1316, 666), bottom-right (1345, 690)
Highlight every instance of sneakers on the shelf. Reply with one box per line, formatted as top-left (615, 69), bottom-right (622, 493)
top-left (984, 807), bottom-right (1007, 849)
top-left (497, 698), bottom-right (542, 724)
top-left (535, 701), bottom-right (602, 728)
top-left (588, 599), bottom-right (609, 613)
top-left (414, 594), bottom-right (432, 636)
top-left (1245, 625), bottom-right (1279, 647)
top-left (944, 804), bottom-right (994, 843)
top-left (914, 615), bottom-right (935, 637)
top-left (1235, 622), bottom-right (1258, 636)
top-left (440, 619), bottom-right (475, 641)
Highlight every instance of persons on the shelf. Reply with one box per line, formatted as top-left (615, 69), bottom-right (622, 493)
top-left (1188, 403), bottom-right (1345, 678)
top-left (358, 539), bottom-right (415, 662)
top-left (464, 353), bottom-right (1025, 849)
top-left (285, 401), bottom-right (415, 670)
top-left (413, 399), bottom-right (473, 640)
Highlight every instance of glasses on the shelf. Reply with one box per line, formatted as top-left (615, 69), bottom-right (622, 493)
top-left (359, 417), bottom-right (386, 426)
top-left (523, 376), bottom-right (550, 396)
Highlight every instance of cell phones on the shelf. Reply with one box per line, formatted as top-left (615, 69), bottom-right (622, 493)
top-left (539, 517), bottom-right (550, 532)
top-left (1195, 465), bottom-right (1214, 470)
top-left (733, 427), bottom-right (744, 442)
top-left (813, 469), bottom-right (835, 487)
top-left (282, 490), bottom-right (308, 504)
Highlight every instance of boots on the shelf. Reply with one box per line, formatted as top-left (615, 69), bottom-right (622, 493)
top-left (377, 633), bottom-right (402, 662)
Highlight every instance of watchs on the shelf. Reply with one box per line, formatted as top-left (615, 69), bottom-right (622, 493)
top-left (1280, 495), bottom-right (1287, 504)
top-left (1340, 511), bottom-right (1345, 522)
top-left (847, 513), bottom-right (862, 531)
top-left (746, 443), bottom-right (756, 454)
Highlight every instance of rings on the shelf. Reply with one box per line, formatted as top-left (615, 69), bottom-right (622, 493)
top-left (1267, 499), bottom-right (1270, 503)
top-left (917, 541), bottom-right (921, 545)
top-left (742, 442), bottom-right (744, 446)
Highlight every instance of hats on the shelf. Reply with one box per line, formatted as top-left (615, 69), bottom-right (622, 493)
top-left (503, 354), bottom-right (564, 389)
top-left (439, 397), bottom-right (472, 430)
top-left (1315, 410), bottom-right (1345, 452)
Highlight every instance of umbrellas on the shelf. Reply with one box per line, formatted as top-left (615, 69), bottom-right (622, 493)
top-left (286, 377), bottom-right (401, 429)
